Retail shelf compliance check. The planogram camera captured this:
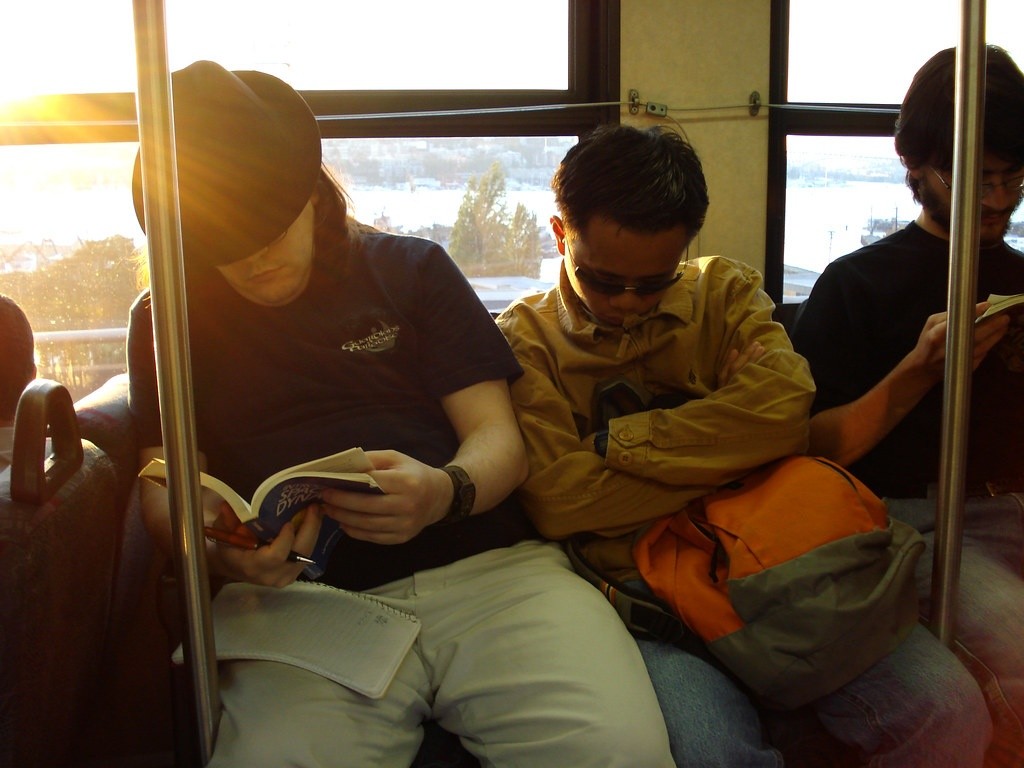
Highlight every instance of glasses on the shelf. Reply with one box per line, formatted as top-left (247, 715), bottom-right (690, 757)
top-left (564, 233), bottom-right (690, 296)
top-left (929, 166), bottom-right (1023, 202)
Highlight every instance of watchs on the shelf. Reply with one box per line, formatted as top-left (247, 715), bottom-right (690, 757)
top-left (440, 465), bottom-right (475, 525)
top-left (592, 429), bottom-right (608, 457)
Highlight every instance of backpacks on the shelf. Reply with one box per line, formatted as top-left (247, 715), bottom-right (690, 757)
top-left (592, 378), bottom-right (931, 707)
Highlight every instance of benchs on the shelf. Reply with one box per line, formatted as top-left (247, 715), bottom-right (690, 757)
top-left (0, 290), bottom-right (198, 767)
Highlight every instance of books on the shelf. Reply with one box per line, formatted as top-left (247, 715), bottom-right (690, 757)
top-left (975, 294), bottom-right (1024, 324)
top-left (138, 447), bottom-right (386, 579)
top-left (170, 581), bottom-right (422, 699)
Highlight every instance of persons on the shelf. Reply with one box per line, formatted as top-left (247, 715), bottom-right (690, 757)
top-left (0, 296), bottom-right (37, 472)
top-left (791, 46), bottom-right (1024, 768)
top-left (493, 122), bottom-right (992, 768)
top-left (127, 60), bottom-right (675, 767)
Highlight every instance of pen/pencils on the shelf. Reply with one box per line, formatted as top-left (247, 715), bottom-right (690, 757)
top-left (203, 527), bottom-right (317, 565)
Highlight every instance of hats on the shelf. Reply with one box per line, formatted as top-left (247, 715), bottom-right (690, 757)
top-left (131, 60), bottom-right (323, 267)
